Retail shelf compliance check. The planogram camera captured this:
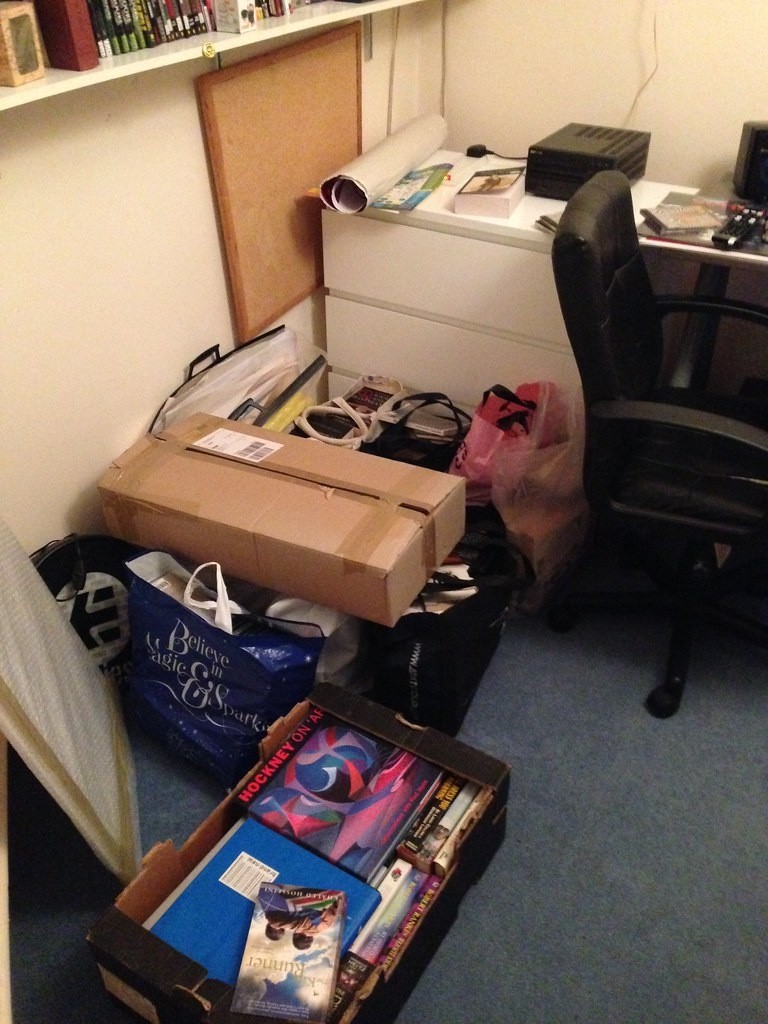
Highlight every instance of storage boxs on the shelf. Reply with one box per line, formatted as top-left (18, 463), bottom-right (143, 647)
top-left (148, 571), bottom-right (205, 606)
top-left (215, 0), bottom-right (257, 34)
top-left (0, 1), bottom-right (45, 87)
top-left (97, 412), bottom-right (467, 627)
top-left (85, 679), bottom-right (512, 1024)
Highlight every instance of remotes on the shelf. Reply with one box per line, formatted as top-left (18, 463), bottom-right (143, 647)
top-left (711, 207), bottom-right (763, 246)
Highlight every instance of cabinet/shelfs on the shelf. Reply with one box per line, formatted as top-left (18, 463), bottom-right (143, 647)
top-left (322, 149), bottom-right (701, 412)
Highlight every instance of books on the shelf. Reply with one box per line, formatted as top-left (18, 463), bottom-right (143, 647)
top-left (140, 705), bottom-right (494, 1024)
top-left (83, 1), bottom-right (216, 58)
top-left (455, 165), bottom-right (526, 219)
top-left (255, 0), bottom-right (311, 22)
top-left (346, 388), bottom-right (470, 436)
top-left (640, 205), bottom-right (723, 236)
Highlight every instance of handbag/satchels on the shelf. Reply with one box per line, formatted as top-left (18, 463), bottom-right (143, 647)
top-left (149, 324), bottom-right (328, 436)
top-left (494, 431), bottom-right (589, 617)
top-left (289, 374), bottom-right (473, 473)
top-left (368, 533), bottom-right (536, 734)
top-left (120, 551), bottom-right (325, 782)
top-left (449, 380), bottom-right (572, 504)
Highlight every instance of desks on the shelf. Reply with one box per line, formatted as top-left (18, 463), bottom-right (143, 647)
top-left (631, 184), bottom-right (768, 391)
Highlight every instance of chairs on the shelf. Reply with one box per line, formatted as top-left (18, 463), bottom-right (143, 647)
top-left (551, 170), bottom-right (768, 719)
